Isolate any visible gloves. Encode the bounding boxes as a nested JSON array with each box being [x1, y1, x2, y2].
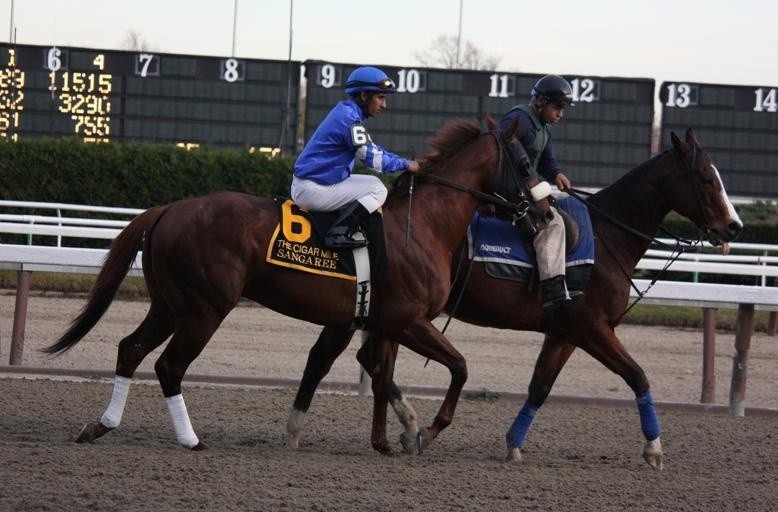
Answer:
[[518, 157, 530, 177]]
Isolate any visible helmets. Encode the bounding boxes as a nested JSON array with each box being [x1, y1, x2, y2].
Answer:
[[343, 66, 397, 94], [531, 76, 573, 102]]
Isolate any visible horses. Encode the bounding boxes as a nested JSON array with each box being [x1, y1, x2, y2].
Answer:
[[37, 114, 554, 452], [285, 125, 745, 474]]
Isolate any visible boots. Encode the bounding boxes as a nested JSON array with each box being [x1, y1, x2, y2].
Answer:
[[541, 277, 584, 312], [324, 200, 370, 252]]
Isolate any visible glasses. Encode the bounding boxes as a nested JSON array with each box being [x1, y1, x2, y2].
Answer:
[[378, 79, 397, 90]]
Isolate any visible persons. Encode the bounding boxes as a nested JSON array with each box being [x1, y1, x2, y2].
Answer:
[[482, 76, 587, 317], [290, 66, 422, 254]]
